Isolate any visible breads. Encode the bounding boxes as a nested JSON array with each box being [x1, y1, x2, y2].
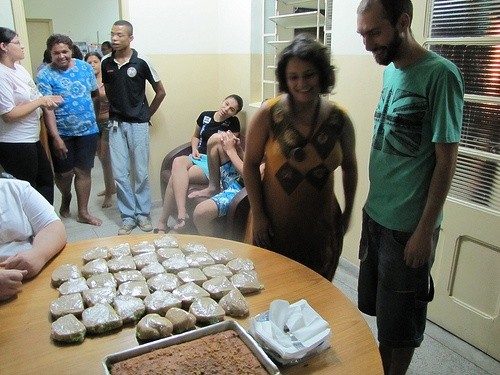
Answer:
[[112, 329, 271, 375], [49, 237, 263, 343]]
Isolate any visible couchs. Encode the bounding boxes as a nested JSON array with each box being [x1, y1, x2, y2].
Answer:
[[160, 141, 250, 242]]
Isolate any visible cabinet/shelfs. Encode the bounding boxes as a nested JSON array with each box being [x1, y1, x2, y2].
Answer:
[[246, 0, 333, 144]]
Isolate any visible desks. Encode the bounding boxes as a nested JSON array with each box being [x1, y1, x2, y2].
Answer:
[[0, 234, 385, 375]]
